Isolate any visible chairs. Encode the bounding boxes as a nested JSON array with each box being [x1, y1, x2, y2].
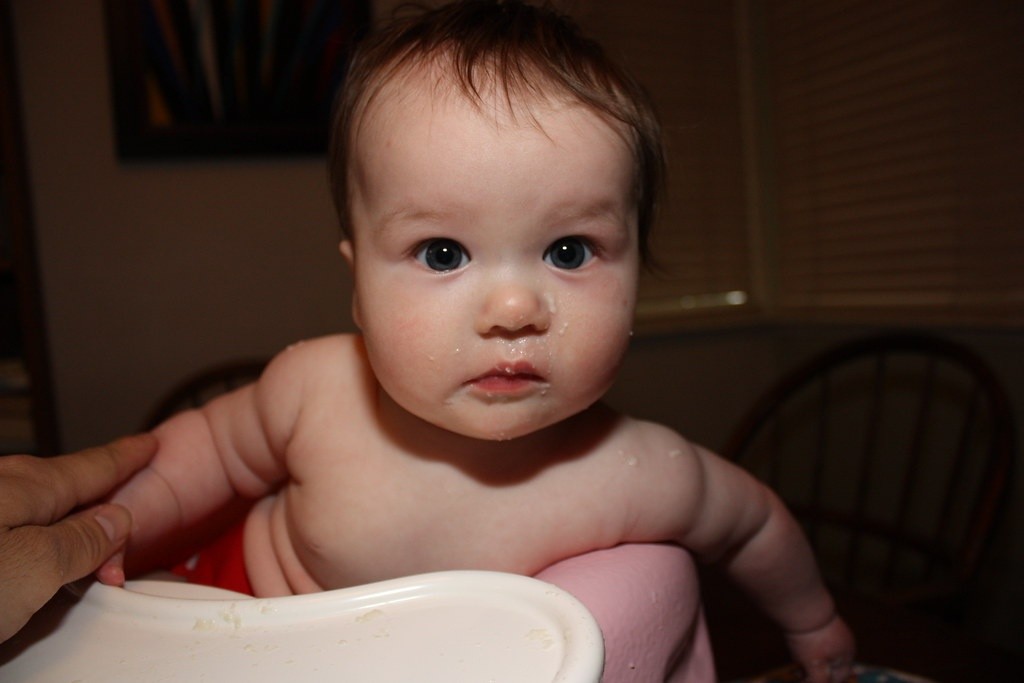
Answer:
[[718, 327, 1017, 616]]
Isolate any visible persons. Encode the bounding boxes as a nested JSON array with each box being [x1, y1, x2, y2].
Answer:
[[0, 433, 702, 683], [94, 0, 856, 683]]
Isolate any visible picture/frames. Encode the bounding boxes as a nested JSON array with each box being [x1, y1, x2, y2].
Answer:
[[103, 0, 369, 159]]
